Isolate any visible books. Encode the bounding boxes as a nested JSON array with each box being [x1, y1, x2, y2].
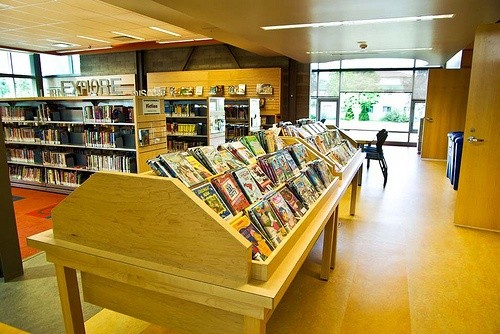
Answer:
[[224, 100, 250, 142], [162, 99, 209, 153], [0, 98, 137, 188], [146, 118, 358, 261], [158, 84, 273, 97]]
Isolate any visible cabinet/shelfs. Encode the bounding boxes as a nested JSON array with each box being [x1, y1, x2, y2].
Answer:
[[26, 135, 341, 333], [0, 93, 167, 195], [0, 116, 24, 283], [265, 124, 368, 271], [162, 96, 226, 153], [260, 112, 282, 131], [222, 97, 262, 143]]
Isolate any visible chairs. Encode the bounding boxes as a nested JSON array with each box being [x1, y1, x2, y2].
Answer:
[[354, 128, 389, 188]]
